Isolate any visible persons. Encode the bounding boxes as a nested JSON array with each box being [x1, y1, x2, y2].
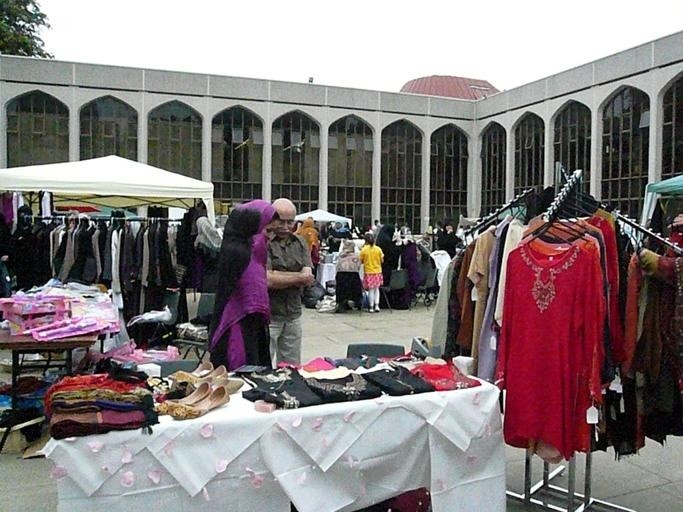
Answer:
[[208, 199, 316, 372], [360, 234, 384, 313], [266, 197, 315, 367], [298, 218, 320, 279], [194, 216, 223, 292], [437, 223, 459, 258]]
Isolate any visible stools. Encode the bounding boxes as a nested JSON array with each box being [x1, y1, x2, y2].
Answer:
[[172, 339, 206, 366]]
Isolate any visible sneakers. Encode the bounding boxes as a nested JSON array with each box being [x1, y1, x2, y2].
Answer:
[[369, 307, 380, 312]]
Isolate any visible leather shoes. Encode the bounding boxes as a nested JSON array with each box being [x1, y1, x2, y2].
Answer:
[[158, 361, 244, 420]]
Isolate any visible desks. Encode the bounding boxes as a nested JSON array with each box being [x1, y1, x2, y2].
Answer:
[[314, 263, 365, 289], [51, 358, 506, 512], [0, 332, 98, 411]]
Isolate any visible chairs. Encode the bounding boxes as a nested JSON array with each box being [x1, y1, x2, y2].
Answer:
[[414, 269, 438, 308], [133, 291, 180, 348], [379, 270, 412, 313], [348, 344, 405, 359]]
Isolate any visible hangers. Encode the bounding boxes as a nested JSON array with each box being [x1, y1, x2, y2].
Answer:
[[527, 173, 598, 247], [19, 213, 180, 233], [619, 215, 671, 258], [461, 193, 527, 251]]
